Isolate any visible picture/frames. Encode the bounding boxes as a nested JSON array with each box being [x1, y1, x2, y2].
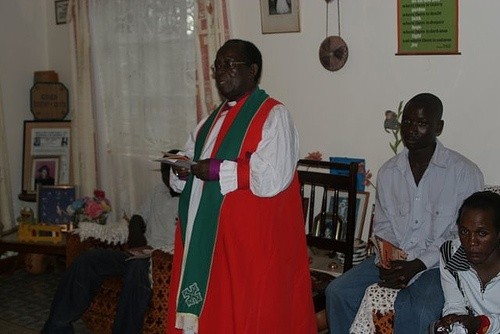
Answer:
[[22, 120, 72, 199], [318, 190, 371, 246], [29, 83, 70, 121], [36, 184, 75, 233], [261, 0, 301, 36], [28, 154, 61, 194], [54, 0, 70, 26]]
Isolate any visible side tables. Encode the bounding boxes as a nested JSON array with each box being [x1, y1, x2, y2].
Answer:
[[0, 225, 73, 281]]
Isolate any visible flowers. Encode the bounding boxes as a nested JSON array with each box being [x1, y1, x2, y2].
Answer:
[[66, 190, 112, 224]]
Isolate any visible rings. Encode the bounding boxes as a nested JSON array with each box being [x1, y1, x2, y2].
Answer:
[[176, 172, 178, 176]]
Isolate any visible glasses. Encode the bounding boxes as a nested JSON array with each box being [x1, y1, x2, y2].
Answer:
[[210, 61, 246, 75]]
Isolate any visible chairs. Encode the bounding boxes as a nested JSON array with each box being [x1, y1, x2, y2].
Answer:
[[349, 184, 500, 334], [294, 157, 358, 330]]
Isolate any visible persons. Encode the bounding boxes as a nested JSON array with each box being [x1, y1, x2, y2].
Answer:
[[430, 190, 500, 334], [325, 93, 484, 334], [34, 165, 54, 192], [41, 149, 182, 334], [165, 38, 317, 334]]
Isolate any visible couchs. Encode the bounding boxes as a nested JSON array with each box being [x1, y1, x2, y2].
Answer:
[[64, 186, 178, 334]]
